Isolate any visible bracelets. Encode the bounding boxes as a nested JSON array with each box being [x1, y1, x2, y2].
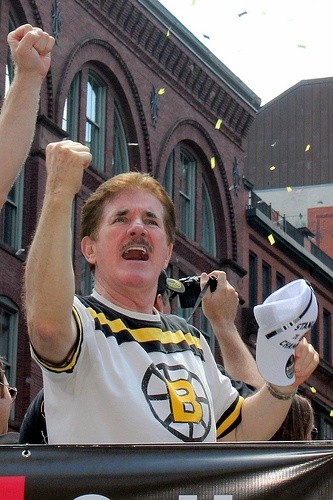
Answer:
[[268, 382, 299, 400]]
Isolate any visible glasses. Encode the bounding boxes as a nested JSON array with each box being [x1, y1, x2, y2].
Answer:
[[0, 382, 18, 402]]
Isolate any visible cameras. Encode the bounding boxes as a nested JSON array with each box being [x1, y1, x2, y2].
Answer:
[[178, 275, 217, 308]]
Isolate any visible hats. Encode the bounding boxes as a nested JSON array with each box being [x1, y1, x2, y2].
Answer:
[[253, 278, 318, 387], [157, 269, 185, 294]]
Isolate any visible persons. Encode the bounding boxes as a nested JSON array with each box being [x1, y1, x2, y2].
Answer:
[[153, 268, 266, 398], [19, 388, 48, 444], [0, 361, 16, 436], [0, 22, 55, 208], [22, 139, 320, 445], [270, 395, 315, 441]]
